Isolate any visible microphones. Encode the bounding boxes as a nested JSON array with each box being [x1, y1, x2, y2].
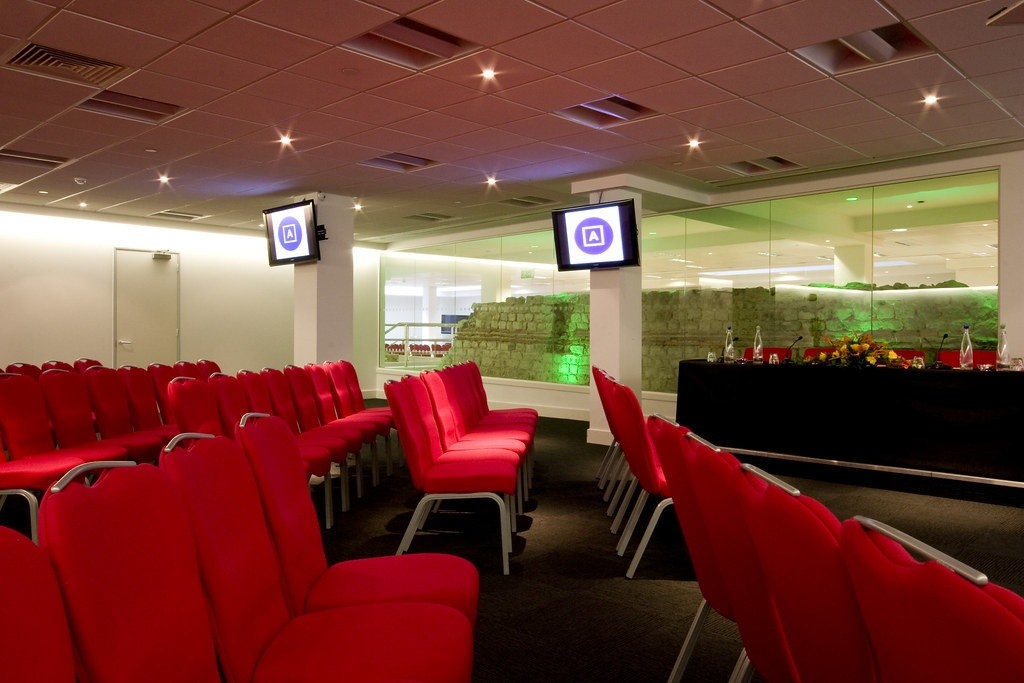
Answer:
[[719, 337, 739, 363], [932, 334, 952, 370], [782, 336, 803, 364]]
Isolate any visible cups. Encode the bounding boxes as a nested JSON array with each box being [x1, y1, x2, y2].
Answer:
[[707, 352, 717, 363], [1011, 358, 1023, 371], [769, 353, 779, 364]]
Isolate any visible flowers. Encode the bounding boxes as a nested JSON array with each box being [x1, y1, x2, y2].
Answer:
[[803, 331, 910, 370]]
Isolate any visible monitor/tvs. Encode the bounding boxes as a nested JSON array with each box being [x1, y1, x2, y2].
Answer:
[[551, 198, 642, 271], [263, 199, 328, 267]]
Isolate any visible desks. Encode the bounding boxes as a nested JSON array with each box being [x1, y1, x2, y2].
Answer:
[[677, 358, 1024, 510]]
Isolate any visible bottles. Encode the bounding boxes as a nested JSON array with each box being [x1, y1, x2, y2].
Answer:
[[996, 324, 1010, 371], [724, 326, 735, 364], [959, 324, 973, 370], [752, 326, 764, 364]]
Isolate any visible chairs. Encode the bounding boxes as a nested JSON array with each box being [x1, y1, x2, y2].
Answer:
[[1, 358, 1023, 681]]
